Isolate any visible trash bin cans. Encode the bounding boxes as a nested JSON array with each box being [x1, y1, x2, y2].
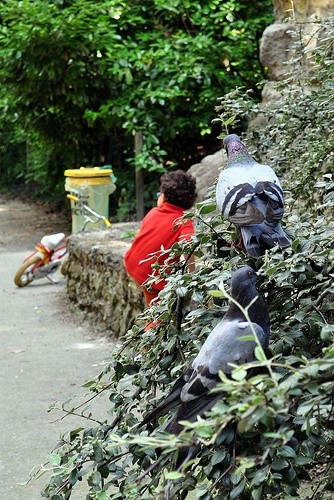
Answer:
[[64, 165, 117, 234]]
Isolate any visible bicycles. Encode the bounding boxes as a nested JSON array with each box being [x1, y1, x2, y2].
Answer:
[[13, 194, 111, 287]]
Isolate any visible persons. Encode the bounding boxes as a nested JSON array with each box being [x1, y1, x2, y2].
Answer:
[[122, 170, 198, 334]]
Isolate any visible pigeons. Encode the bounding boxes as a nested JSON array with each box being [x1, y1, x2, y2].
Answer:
[[136, 267, 275, 437], [215, 134, 291, 259]]
